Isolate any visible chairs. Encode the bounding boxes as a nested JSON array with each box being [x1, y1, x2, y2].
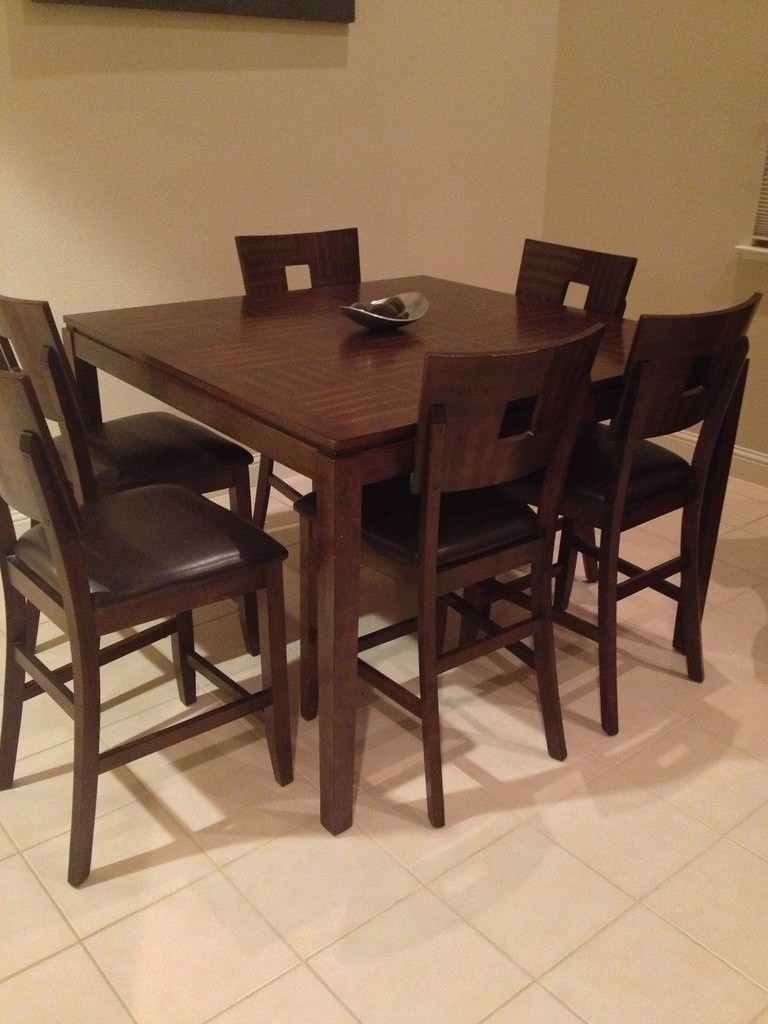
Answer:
[[0, 370, 291, 886], [292, 323, 605, 829], [0, 297, 261, 657], [231, 226, 362, 529], [506, 237, 636, 586], [478, 293, 762, 736]]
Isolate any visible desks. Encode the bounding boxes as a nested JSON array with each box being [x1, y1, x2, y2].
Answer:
[[61, 273, 640, 837]]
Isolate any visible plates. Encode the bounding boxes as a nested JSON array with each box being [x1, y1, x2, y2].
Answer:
[[339, 292, 429, 330]]
